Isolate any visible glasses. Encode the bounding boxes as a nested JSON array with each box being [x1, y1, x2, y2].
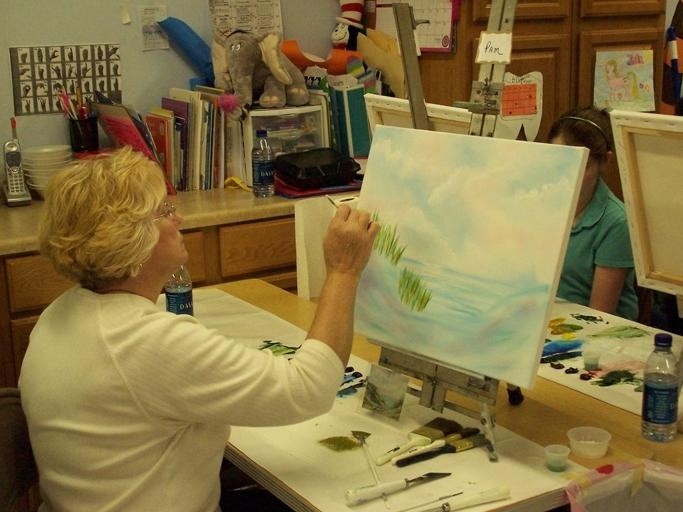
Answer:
[[150, 199, 176, 222]]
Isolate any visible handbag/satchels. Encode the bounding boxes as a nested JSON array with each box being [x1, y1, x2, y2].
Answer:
[[276, 147, 355, 188]]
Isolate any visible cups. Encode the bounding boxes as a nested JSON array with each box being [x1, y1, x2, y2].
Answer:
[[543, 443, 570, 472], [582, 352, 602, 370]]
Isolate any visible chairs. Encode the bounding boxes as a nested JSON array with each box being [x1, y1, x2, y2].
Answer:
[[0, 387, 41, 510]]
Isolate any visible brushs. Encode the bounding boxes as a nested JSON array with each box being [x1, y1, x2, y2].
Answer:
[[377, 417, 488, 467]]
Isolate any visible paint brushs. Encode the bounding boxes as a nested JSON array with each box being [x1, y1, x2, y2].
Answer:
[[351, 430, 388, 501], [325, 195, 339, 209]]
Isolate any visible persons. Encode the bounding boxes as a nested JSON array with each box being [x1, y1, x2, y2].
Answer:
[[539, 106, 644, 322], [15, 141, 382, 512]]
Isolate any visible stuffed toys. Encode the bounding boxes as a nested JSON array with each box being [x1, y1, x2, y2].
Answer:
[[324, 0, 367, 65], [210, 24, 311, 108]]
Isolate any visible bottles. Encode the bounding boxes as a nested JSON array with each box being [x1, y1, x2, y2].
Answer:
[[251, 130, 275, 197], [163, 265, 193, 319], [640, 332, 679, 443]]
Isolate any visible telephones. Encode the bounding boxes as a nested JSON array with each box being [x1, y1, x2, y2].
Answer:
[[0, 116, 32, 207]]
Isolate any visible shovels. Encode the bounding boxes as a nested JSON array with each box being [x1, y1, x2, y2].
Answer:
[[347, 471, 453, 505]]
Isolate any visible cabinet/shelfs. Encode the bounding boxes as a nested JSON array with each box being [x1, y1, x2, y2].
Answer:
[[379, 1, 667, 201], [0, 186, 301, 392]]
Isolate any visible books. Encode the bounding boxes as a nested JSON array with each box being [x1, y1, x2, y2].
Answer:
[[97, 78, 385, 199]]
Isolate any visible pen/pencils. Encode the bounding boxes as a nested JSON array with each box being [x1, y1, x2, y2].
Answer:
[[58, 82, 91, 152]]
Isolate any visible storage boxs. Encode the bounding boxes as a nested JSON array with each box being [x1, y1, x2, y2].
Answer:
[[228, 94, 331, 194]]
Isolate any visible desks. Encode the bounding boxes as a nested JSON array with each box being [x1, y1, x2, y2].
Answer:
[[310, 290, 681, 477], [148, 276, 657, 512]]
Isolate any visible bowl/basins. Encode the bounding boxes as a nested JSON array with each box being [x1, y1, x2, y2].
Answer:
[[20, 143, 74, 200], [566, 425, 611, 461]]
